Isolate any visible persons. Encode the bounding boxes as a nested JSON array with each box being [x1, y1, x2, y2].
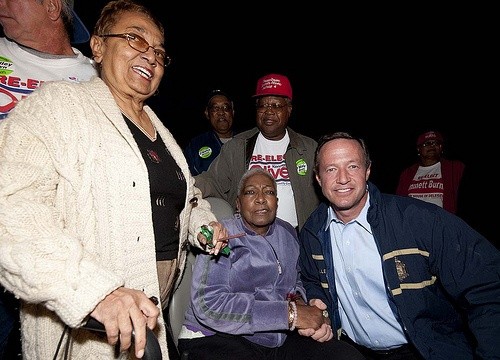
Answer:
[[0, 0, 102, 123], [300, 131, 500, 360], [0, 0, 230, 360], [194, 74, 319, 239], [396, 131, 475, 217], [182, 89, 235, 176], [177, 169, 365, 360]]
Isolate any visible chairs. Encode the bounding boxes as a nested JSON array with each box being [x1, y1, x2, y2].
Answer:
[[169, 196, 233, 345]]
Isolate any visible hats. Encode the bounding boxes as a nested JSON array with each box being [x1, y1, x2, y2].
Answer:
[[416, 132, 443, 146], [253, 74, 293, 100], [63, 0, 91, 44]]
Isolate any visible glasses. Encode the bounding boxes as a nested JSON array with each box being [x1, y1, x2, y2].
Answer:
[[255, 103, 289, 113], [99, 32, 172, 68], [421, 141, 441, 147], [208, 105, 234, 113]]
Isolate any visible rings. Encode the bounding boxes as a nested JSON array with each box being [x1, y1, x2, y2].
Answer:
[[323, 310, 328, 317]]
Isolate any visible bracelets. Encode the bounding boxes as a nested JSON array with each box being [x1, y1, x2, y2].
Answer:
[[288, 302, 297, 331]]
